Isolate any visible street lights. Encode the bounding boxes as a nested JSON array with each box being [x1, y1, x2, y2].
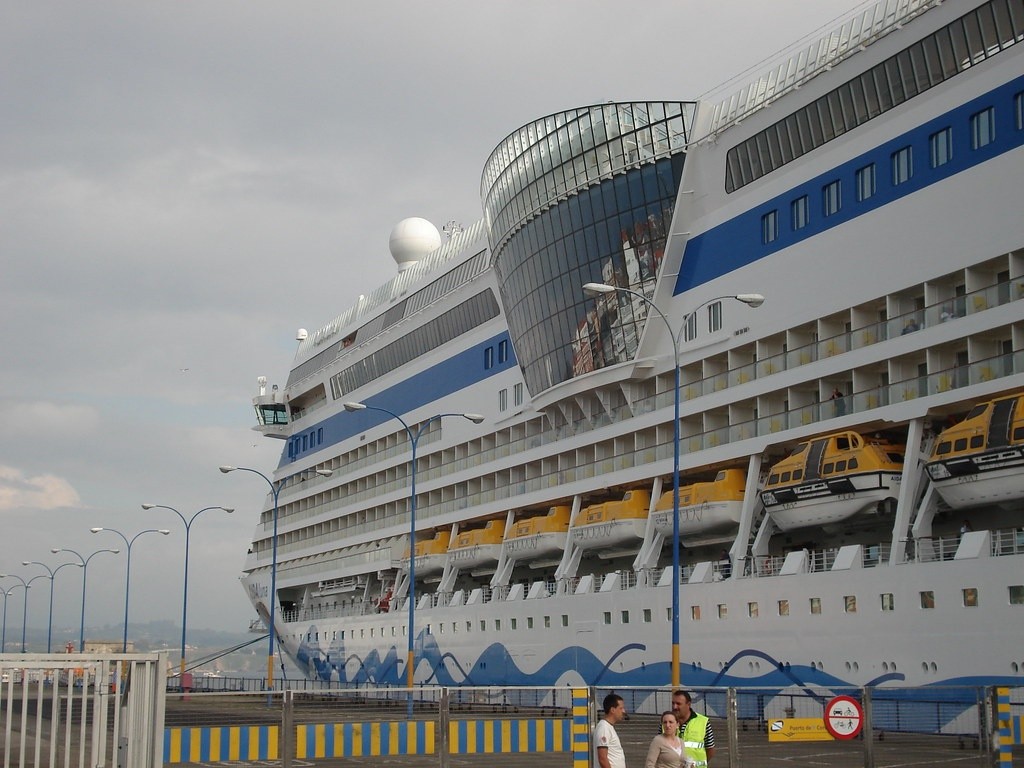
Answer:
[[343, 400, 486, 719], [0, 584, 32, 653], [90, 527, 170, 654], [22, 560, 84, 653], [52, 548, 120, 655], [1, 573, 54, 653], [218, 465, 333, 703], [141, 501, 235, 703], [581, 281, 764, 722]]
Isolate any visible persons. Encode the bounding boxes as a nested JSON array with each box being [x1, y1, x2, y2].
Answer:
[[645, 711, 694, 768], [902, 319, 919, 335], [941, 307, 959, 321], [593, 694, 626, 768], [830, 387, 846, 417], [658, 690, 716, 768]]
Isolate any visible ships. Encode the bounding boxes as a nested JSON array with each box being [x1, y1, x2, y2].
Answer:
[[236, 3, 1024, 749]]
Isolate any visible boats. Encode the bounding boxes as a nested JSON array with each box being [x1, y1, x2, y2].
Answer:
[[501, 506, 580, 572], [763, 432, 907, 536], [651, 466, 748, 548], [568, 488, 653, 559], [401, 530, 451, 585], [921, 392, 1024, 513], [445, 516, 507, 579]]
